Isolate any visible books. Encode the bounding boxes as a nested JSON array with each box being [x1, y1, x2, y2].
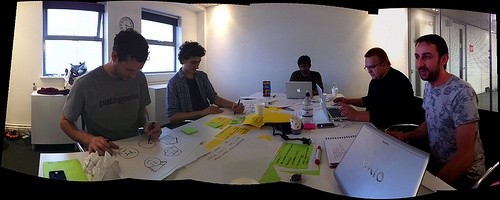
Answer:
[[325, 135, 357, 168]]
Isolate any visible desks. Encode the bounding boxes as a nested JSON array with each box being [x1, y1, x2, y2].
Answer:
[[144, 83, 168, 123], [38, 92, 457, 199], [30, 88, 83, 151]]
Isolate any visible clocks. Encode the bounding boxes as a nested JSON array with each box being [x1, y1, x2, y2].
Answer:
[[119, 17, 134, 32]]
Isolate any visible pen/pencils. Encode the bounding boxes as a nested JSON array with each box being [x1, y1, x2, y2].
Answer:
[[148, 122, 155, 142], [99, 136, 112, 156], [234, 99, 240, 114]]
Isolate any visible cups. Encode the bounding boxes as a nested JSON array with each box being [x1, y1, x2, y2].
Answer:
[[253, 103, 265, 116], [289, 112, 301, 135]]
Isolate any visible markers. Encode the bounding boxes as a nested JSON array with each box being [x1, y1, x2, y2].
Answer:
[[315, 146, 321, 164]]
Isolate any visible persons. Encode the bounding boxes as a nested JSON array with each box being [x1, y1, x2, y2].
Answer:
[[166, 40, 245, 128], [64, 68, 70, 89], [386, 34, 485, 189], [59, 28, 162, 157], [333, 48, 416, 132], [289, 55, 324, 96]]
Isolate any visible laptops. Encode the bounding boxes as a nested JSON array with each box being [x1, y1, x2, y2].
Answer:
[[334, 123, 456, 200], [285, 82, 312, 99], [316, 84, 352, 121]]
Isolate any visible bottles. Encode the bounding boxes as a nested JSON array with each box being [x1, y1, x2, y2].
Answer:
[[302, 92, 313, 124], [331, 81, 339, 96]]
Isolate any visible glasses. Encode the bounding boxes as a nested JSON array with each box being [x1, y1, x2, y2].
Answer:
[[364, 60, 385, 72]]
